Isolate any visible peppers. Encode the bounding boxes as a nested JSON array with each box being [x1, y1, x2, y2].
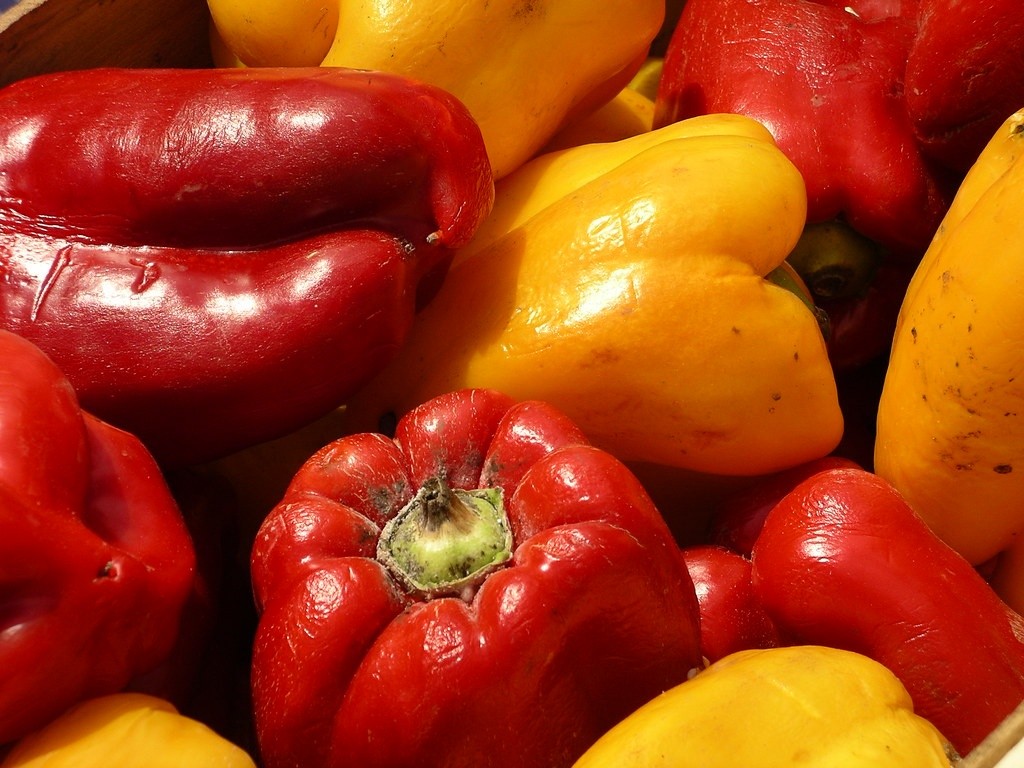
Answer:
[[0, 0, 1024, 768]]
